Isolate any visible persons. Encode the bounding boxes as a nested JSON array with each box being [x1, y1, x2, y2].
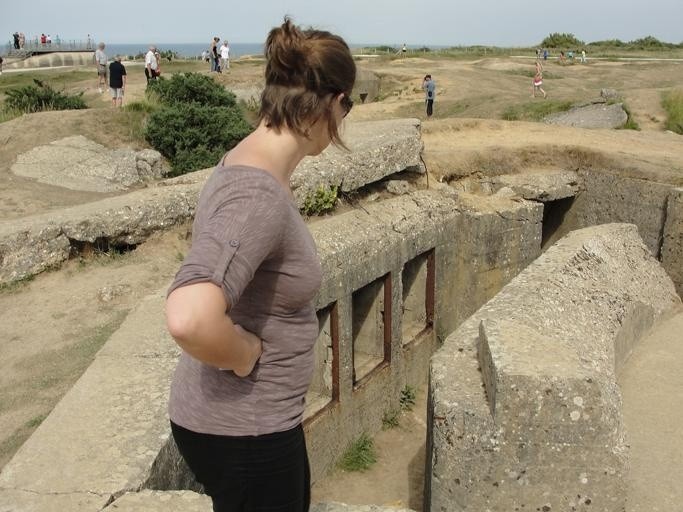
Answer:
[[144, 46, 157, 83], [95, 43, 109, 93], [529, 60, 547, 98], [536, 45, 587, 65], [109, 55, 127, 106], [200, 35, 230, 72], [155, 51, 161, 79], [163, 14, 358, 512], [402, 43, 407, 58], [0, 31, 94, 74], [422, 74, 436, 120]]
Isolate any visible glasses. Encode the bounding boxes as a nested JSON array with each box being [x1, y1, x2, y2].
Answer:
[[322, 84, 353, 118]]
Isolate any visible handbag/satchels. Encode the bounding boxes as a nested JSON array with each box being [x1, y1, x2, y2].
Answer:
[[534, 78, 543, 86], [429, 92, 432, 97]]
